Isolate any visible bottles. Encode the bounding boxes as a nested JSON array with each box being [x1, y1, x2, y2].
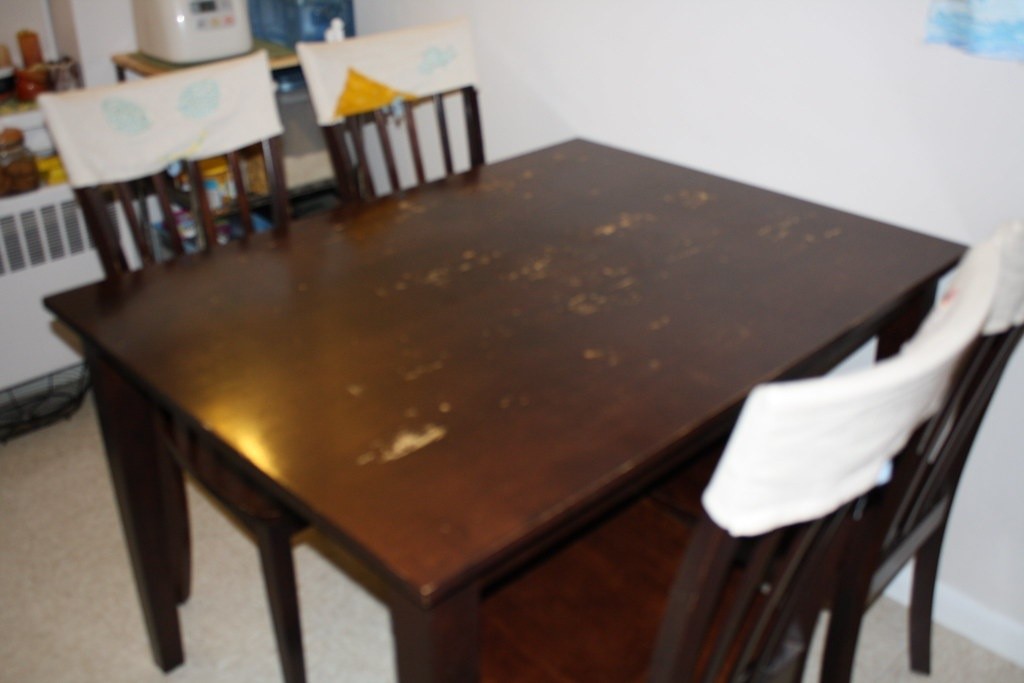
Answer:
[[0, 132, 38, 197]]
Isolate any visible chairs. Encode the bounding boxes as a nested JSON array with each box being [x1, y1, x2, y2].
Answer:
[[297, 17, 484, 202], [817, 224, 1024, 683], [36, 49, 360, 683], [481, 333, 880, 682]]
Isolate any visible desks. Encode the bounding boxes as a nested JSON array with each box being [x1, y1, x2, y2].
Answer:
[[111, 40, 300, 82], [44, 139, 968, 682]]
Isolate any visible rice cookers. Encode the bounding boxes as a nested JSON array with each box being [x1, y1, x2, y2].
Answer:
[[130, 0, 252, 64]]
